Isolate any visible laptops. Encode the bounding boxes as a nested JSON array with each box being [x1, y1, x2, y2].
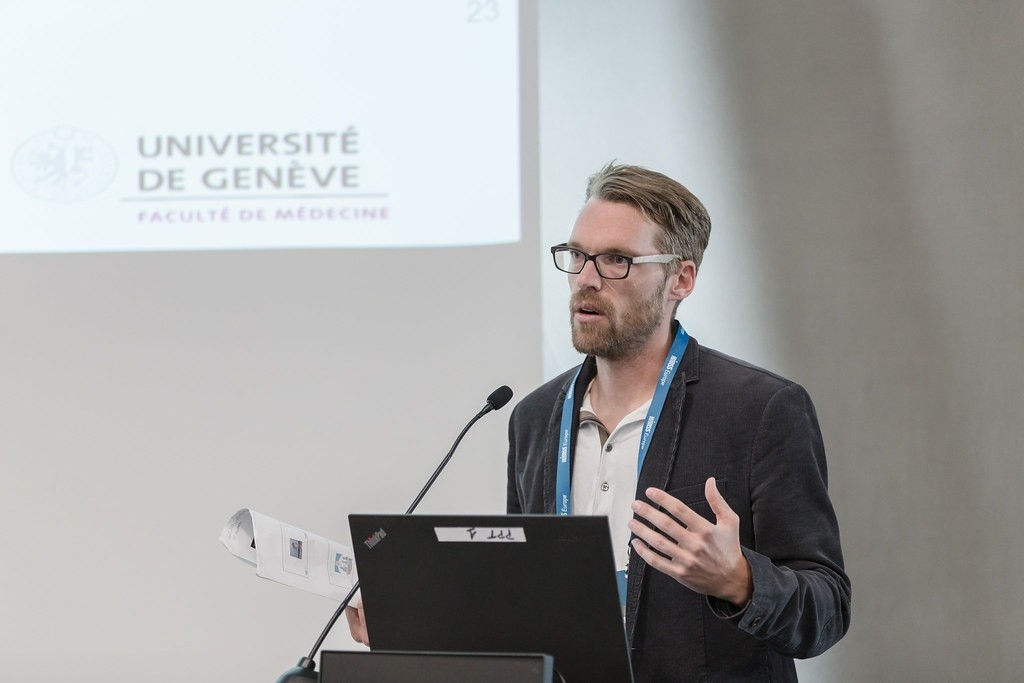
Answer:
[[347, 514, 634, 683]]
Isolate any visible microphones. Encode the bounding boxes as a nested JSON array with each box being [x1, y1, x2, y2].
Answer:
[[278, 385, 514, 683]]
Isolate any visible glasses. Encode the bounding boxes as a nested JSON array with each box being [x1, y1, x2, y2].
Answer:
[[551, 243, 681, 280]]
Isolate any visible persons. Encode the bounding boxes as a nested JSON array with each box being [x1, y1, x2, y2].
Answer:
[[346, 163, 853, 682]]
